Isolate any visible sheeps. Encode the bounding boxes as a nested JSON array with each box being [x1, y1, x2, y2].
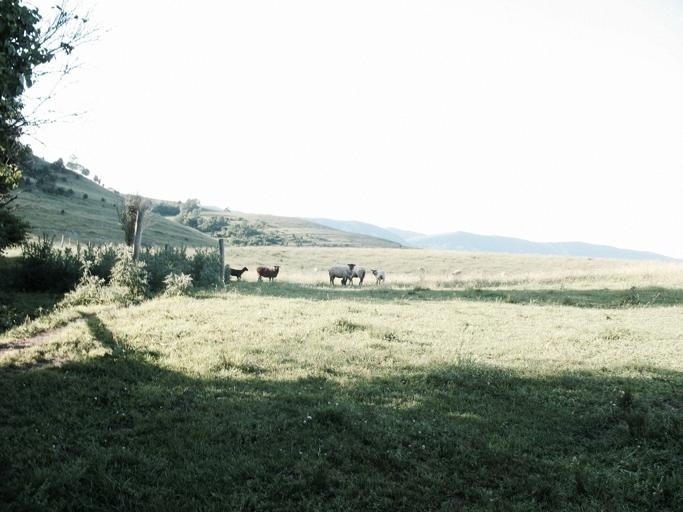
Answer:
[[256, 264, 280, 282], [328, 263, 386, 289], [229, 267, 248, 281]]
